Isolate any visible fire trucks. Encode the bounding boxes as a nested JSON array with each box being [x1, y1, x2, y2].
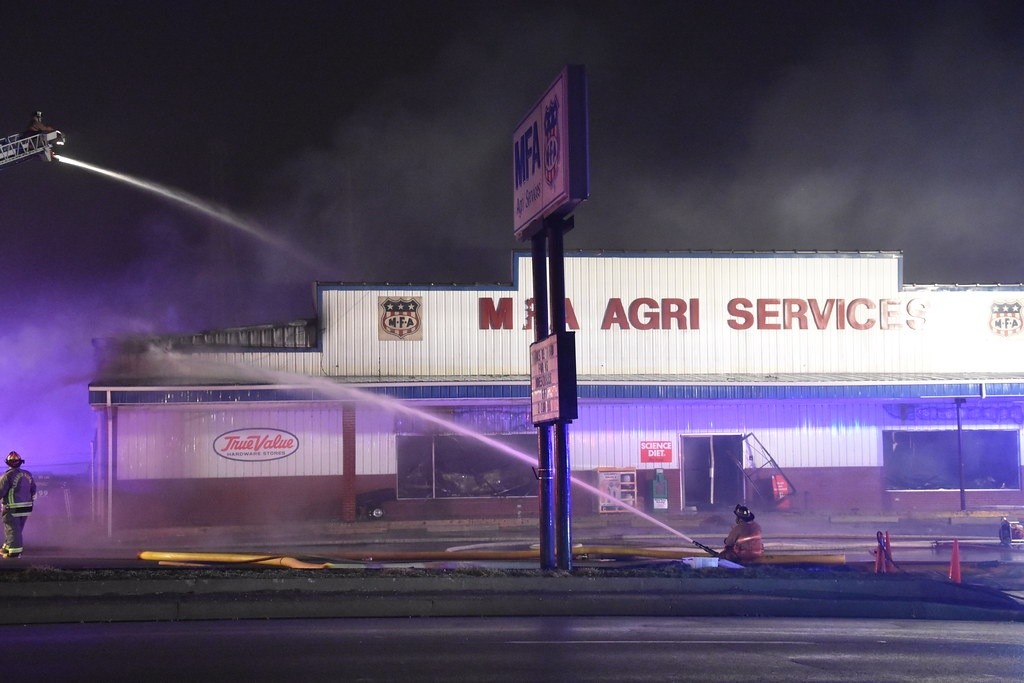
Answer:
[[0, 130, 69, 171]]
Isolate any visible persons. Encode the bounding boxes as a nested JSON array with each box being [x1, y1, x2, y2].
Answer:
[[0, 451, 36, 557], [718, 505, 764, 564]]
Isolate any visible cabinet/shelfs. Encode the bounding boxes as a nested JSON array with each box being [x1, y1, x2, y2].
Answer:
[[592, 467, 638, 514]]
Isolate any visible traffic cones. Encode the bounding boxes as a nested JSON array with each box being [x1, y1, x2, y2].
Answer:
[[947, 536, 961, 584], [875, 532, 885, 576], [883, 530, 900, 575]]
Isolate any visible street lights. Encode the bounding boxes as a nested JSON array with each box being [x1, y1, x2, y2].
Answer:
[[952, 396, 967, 513]]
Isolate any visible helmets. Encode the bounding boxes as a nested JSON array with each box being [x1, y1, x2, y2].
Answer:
[[734, 506, 755, 522], [4, 450, 22, 468]]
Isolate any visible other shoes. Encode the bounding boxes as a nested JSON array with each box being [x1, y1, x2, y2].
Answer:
[[0, 548, 9, 558]]
